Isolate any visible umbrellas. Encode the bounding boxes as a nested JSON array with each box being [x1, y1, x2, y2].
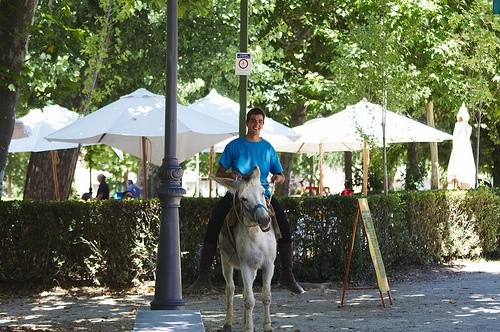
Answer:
[[8, 104, 102, 200], [297, 98, 453, 197], [44, 87, 237, 201], [263, 112, 389, 196], [445, 102, 479, 189], [185, 90, 307, 200]]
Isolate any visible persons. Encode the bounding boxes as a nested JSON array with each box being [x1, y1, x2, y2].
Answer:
[[341, 182, 353, 197], [96, 174, 110, 201], [322, 187, 331, 197], [195, 107, 305, 294], [128, 180, 141, 198], [123, 191, 133, 199]]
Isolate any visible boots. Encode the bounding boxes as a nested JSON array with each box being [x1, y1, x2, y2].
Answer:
[[188, 241, 217, 290], [278, 243, 305, 295]]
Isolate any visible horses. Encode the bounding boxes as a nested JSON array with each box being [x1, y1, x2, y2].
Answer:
[[211, 165, 278, 332]]
